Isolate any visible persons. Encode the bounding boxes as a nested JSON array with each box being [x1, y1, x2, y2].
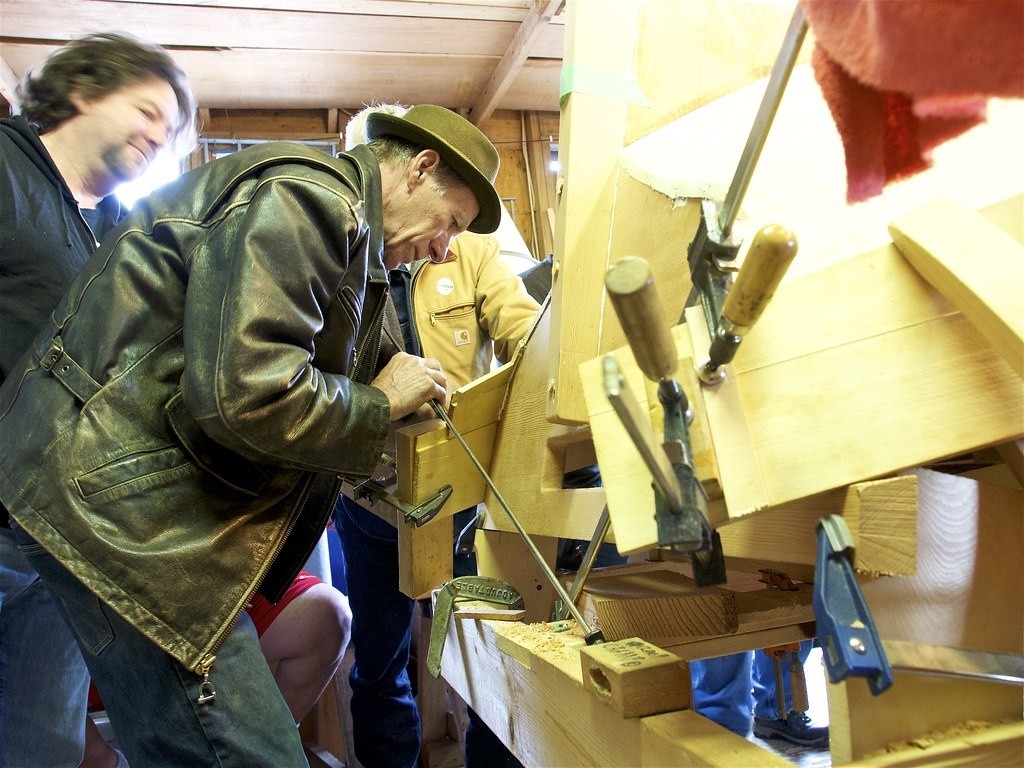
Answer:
[[0, 104, 503, 768], [0, 32, 554, 768]]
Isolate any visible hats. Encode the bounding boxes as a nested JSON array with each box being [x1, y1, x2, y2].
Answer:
[[367, 103, 500, 233]]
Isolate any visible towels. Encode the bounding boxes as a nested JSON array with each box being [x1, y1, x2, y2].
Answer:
[[794, 1, 1024, 205]]
